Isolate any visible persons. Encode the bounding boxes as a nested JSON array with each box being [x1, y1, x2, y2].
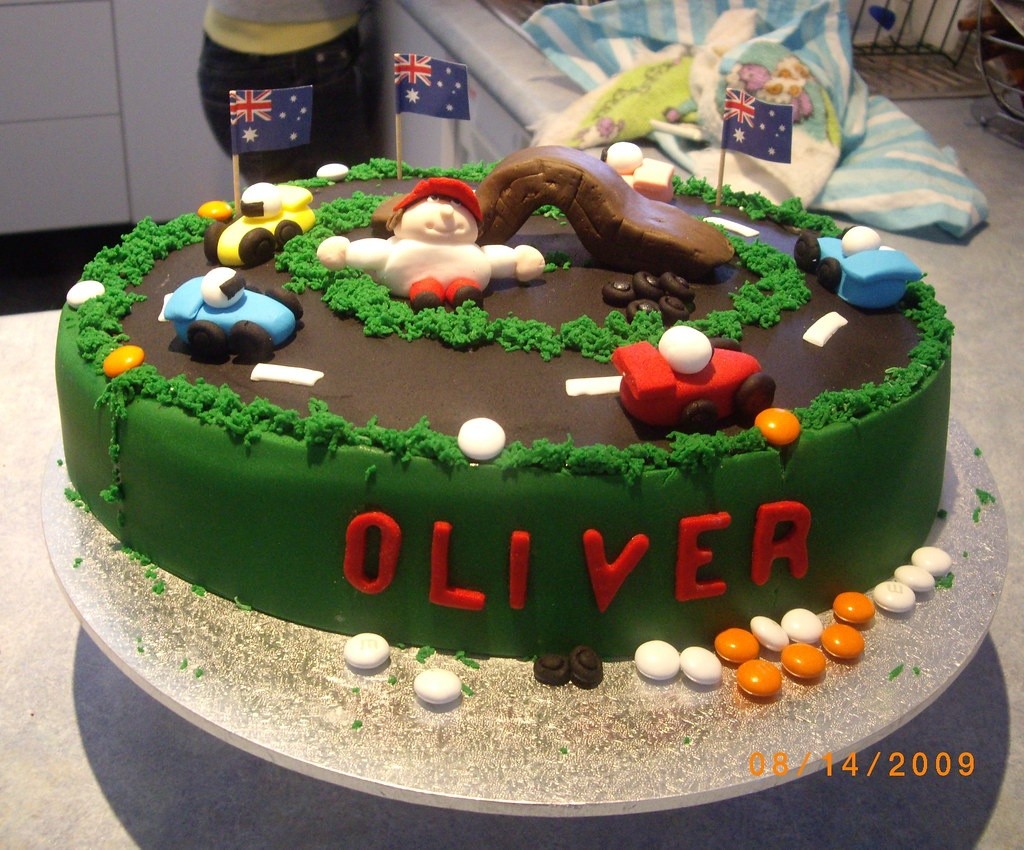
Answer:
[[197, 0, 382, 188], [317, 179, 545, 312]]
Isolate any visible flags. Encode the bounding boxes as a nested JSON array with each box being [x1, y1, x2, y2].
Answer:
[[722, 88, 793, 164], [229, 86, 311, 152], [394, 53, 470, 120]]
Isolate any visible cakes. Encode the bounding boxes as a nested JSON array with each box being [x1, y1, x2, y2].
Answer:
[[52, 50, 955, 702]]
